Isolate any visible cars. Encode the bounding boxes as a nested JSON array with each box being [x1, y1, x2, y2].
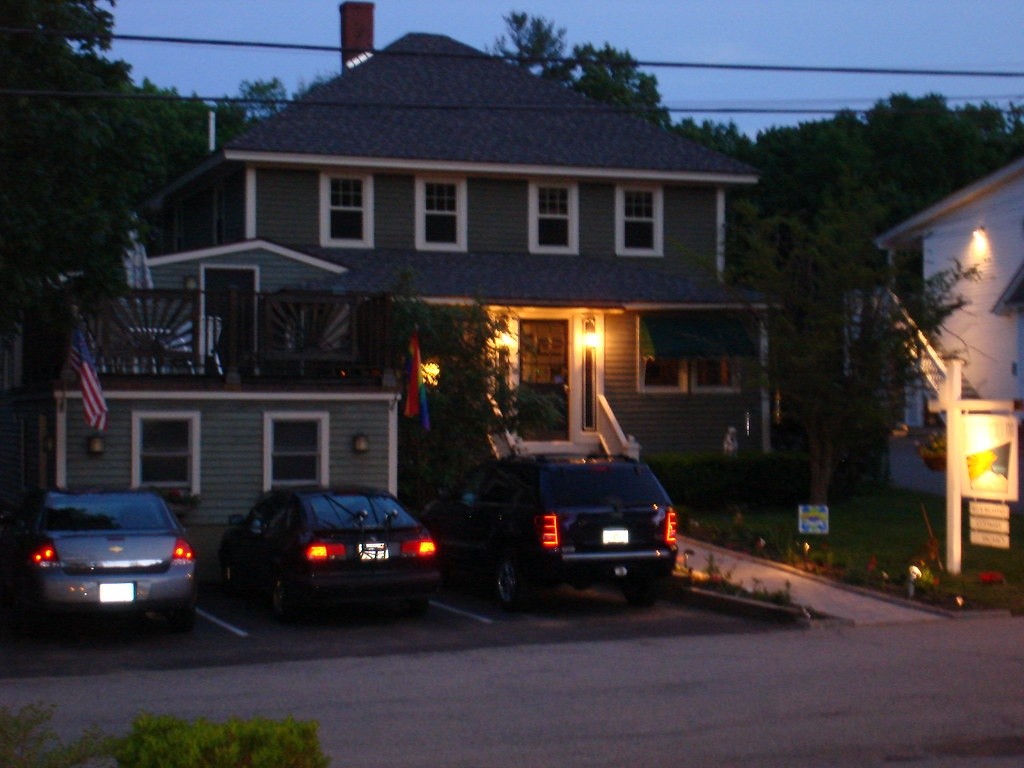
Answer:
[[214, 484, 439, 622], [5, 489, 199, 636]]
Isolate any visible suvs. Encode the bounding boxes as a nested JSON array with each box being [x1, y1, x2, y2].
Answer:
[[417, 454, 676, 616]]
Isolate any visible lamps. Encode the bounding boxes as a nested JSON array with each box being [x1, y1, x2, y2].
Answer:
[[87, 430, 106, 455], [352, 433, 371, 452], [579, 310, 599, 346]]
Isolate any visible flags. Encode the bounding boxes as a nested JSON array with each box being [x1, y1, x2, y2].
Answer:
[[399, 329, 433, 431], [66, 314, 110, 433]]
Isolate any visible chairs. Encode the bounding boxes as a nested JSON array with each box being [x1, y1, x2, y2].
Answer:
[[152, 315, 222, 375]]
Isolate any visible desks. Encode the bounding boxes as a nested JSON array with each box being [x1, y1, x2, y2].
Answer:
[[122, 325, 172, 373]]
